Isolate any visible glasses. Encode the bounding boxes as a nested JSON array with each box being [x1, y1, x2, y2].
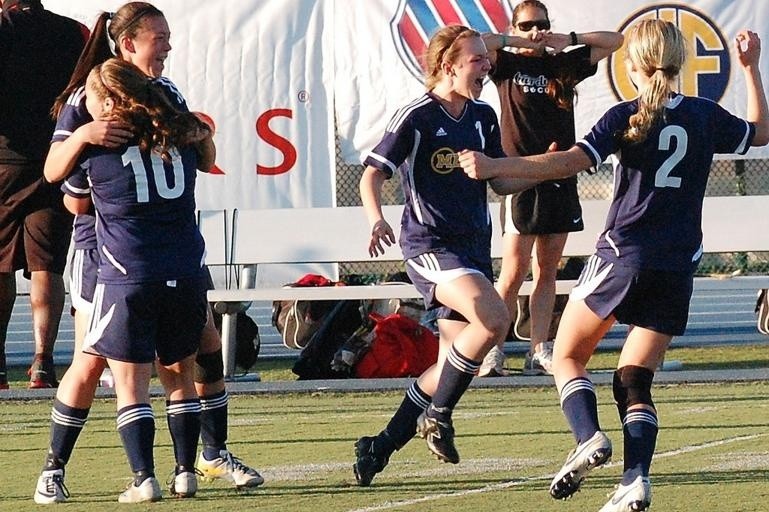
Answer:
[[515, 20, 551, 32]]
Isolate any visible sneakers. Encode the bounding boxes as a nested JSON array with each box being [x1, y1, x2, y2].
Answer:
[[118, 474, 162, 503], [417, 405, 461, 464], [547, 432, 611, 500], [599, 475, 651, 512], [522, 342, 554, 376], [352, 434, 391, 486], [197, 450, 264, 487], [479, 344, 505, 376], [166, 466, 205, 497], [28, 353, 57, 387], [33, 469, 70, 504]]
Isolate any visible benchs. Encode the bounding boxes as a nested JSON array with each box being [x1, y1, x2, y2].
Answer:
[[1, 194, 766, 390]]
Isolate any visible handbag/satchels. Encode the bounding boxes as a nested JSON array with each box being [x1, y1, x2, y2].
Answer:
[[273, 275, 440, 379], [510, 258, 584, 340], [234, 312, 261, 370]]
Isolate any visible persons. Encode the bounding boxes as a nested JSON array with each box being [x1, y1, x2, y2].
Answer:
[[458, 17, 768, 512], [62, 57, 215, 505], [478, 0, 624, 377], [0, 0, 91, 389], [354, 24, 564, 486], [32, 1, 266, 507]]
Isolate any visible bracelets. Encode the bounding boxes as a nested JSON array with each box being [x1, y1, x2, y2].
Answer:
[[500, 34, 507, 49], [569, 31, 579, 46]]
